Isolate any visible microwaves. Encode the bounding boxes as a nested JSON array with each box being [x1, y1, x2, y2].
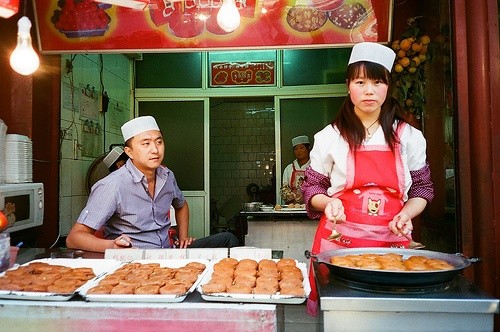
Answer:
[[1, 182, 44, 232]]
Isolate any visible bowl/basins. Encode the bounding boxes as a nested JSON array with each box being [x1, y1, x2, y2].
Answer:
[[242, 201, 274, 212]]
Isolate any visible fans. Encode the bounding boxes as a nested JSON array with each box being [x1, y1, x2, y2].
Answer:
[[246, 183, 259, 202]]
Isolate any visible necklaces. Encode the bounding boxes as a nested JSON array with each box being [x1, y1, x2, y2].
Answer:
[[366, 118, 379, 135]]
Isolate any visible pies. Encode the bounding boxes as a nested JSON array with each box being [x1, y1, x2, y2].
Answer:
[[202, 258, 306, 295], [331, 254, 455, 270], [0, 261, 95, 293], [87, 261, 205, 296]]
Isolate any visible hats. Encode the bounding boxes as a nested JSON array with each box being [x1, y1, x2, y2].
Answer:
[[121, 116, 161, 142], [292, 136, 311, 147], [348, 42, 397, 73], [103, 146, 125, 168]]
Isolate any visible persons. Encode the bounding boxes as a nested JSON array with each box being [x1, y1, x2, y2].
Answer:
[[301, 42, 434, 317], [66, 116, 238, 253], [283, 135, 312, 207]]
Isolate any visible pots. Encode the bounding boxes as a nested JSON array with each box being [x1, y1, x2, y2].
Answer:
[[303, 244, 482, 290]]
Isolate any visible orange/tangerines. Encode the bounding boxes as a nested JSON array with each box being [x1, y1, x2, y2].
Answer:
[[392, 33, 430, 106]]
